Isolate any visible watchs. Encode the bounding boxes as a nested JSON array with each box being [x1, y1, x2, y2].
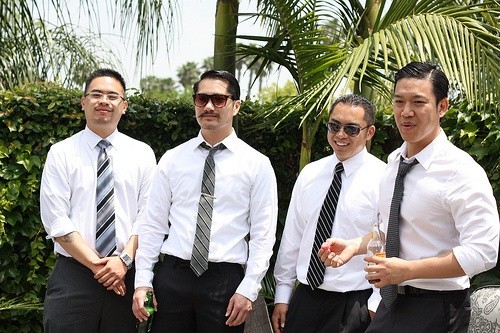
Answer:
[[118, 251, 133, 270]]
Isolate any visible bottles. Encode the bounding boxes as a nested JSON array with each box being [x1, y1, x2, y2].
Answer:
[[366, 223, 386, 284], [136, 292, 155, 333]]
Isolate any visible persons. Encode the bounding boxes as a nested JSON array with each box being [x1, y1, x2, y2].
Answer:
[[273, 93, 388, 333], [133, 68, 278, 333], [40, 68, 157, 333], [318, 61, 500, 333]]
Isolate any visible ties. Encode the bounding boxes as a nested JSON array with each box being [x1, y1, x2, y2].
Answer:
[[305, 163, 345, 291], [95, 139, 118, 260], [189, 143, 228, 279], [379, 153, 418, 309]]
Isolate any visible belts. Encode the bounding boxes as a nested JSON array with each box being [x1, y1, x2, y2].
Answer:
[[398, 285, 469, 297]]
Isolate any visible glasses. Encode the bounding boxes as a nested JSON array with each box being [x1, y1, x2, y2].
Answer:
[[84, 91, 126, 101], [191, 92, 233, 107], [326, 120, 372, 136]]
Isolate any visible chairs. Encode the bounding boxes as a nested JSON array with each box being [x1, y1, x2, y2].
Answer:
[[468, 285, 500, 333]]
